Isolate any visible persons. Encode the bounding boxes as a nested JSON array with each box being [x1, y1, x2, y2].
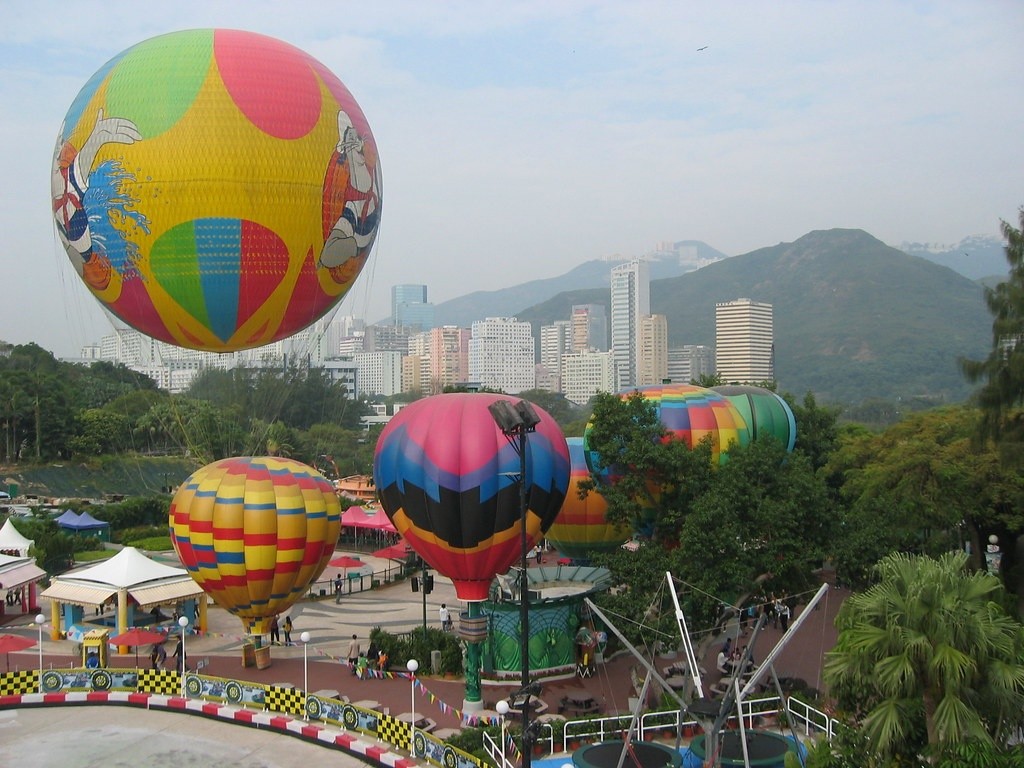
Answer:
[[347, 634, 387, 681], [5, 586, 22, 607], [85, 651, 99, 669], [715, 590, 791, 679], [172, 634, 187, 672], [334, 574, 343, 604], [194, 603, 201, 629], [439, 604, 450, 633], [270, 613, 281, 646], [149, 605, 166, 626], [148, 642, 166, 670], [282, 616, 294, 647]]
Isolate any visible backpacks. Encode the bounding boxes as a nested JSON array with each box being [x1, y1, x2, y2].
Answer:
[[335, 582, 341, 592]]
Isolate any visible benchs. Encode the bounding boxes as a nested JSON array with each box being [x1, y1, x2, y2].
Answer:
[[567, 706, 600, 717], [717, 664, 759, 677], [663, 660, 707, 679], [342, 695, 350, 704], [383, 707, 390, 715], [423, 717, 437, 732], [396, 713, 425, 726], [371, 704, 382, 710]]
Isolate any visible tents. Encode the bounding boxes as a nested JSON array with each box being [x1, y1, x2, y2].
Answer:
[[53, 509, 110, 547], [341, 505, 400, 552]]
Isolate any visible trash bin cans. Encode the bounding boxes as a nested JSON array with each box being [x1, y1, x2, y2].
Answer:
[[431, 650, 442, 676]]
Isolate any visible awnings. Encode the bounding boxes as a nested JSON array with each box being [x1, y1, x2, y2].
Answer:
[[38, 580, 117, 610], [0, 560, 47, 592], [126, 578, 207, 609]]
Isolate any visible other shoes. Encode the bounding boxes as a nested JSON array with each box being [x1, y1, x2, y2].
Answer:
[[336, 602, 341, 605], [352, 671, 355, 676], [750, 626, 755, 630], [761, 627, 765, 631]]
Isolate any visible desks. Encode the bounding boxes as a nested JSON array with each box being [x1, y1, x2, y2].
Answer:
[[667, 678, 685, 689], [728, 660, 752, 673], [270, 682, 294, 689], [567, 690, 594, 716], [314, 689, 341, 700], [673, 661, 697, 675], [432, 728, 463, 740], [352, 700, 379, 710]]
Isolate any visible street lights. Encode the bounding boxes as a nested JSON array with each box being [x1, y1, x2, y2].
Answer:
[[496, 701, 511, 768], [404, 659, 421, 761], [34, 614, 45, 693], [300, 631, 311, 720], [177, 615, 189, 699]]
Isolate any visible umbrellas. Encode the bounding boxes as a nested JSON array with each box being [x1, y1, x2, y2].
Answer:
[[106, 628, 166, 669], [0, 633, 37, 672], [330, 556, 366, 595], [371, 546, 410, 582]]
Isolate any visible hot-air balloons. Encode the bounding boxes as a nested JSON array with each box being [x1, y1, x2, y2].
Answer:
[[541, 433, 637, 565], [583, 378, 753, 556], [709, 384, 798, 490], [370, 391, 572, 721], [165, 453, 343, 670], [45, 25, 386, 474]]
